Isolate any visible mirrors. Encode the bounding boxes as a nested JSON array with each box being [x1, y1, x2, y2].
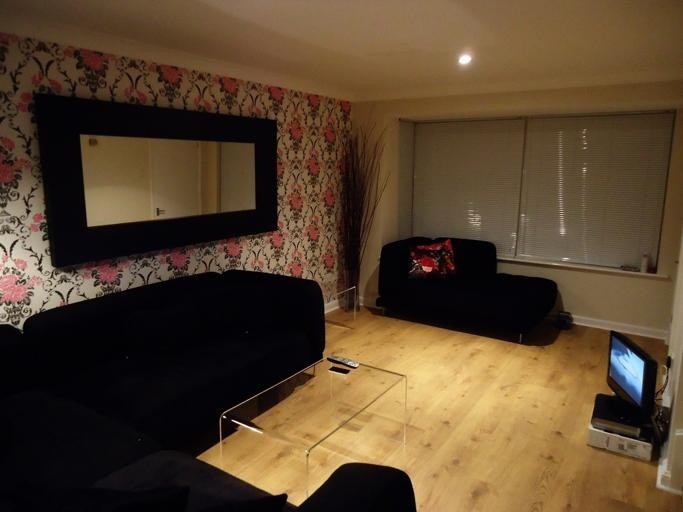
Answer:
[[34, 92, 278, 266]]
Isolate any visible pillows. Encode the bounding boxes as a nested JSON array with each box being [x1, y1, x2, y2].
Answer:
[[408, 240, 458, 282]]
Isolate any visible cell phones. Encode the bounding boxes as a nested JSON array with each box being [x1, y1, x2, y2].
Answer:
[[328, 366, 350, 376]]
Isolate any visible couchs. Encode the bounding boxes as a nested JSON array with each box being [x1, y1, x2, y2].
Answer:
[[374, 238, 575, 345], [0, 269, 418, 510]]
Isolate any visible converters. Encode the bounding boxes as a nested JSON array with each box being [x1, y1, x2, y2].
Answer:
[[666, 355, 672, 369]]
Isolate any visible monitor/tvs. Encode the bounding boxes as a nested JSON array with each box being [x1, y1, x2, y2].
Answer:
[[606, 329, 658, 419]]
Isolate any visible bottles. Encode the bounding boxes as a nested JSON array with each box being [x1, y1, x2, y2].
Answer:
[[640, 255, 648, 273]]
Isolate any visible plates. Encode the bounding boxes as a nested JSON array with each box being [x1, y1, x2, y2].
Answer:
[[623, 267, 639, 272]]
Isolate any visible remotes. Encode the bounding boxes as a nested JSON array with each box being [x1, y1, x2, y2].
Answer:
[[328, 354, 359, 368]]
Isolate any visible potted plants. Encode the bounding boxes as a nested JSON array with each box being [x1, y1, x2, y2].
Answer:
[[337, 124, 391, 313]]
